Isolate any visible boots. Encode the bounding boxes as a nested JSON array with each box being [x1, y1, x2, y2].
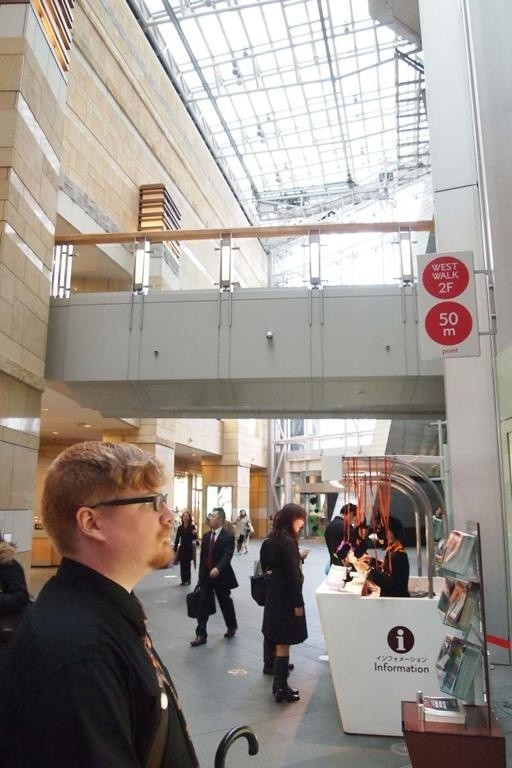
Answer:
[[271, 653, 300, 704]]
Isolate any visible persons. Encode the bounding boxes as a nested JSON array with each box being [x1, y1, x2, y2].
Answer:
[[0, 537, 33, 640], [345, 515, 412, 596], [172, 510, 198, 585], [232, 509, 253, 556], [261, 501, 309, 702], [1, 436, 202, 767], [323, 501, 361, 566], [250, 510, 312, 676], [188, 505, 239, 646]]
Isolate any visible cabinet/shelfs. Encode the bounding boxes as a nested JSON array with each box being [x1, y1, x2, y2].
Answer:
[[400, 519, 508, 768]]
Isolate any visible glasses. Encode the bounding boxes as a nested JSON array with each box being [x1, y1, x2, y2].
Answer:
[[85, 489, 170, 511]]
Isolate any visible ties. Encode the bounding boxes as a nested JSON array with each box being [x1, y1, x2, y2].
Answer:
[[206, 532, 216, 570]]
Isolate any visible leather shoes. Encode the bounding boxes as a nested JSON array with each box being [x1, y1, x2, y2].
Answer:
[[223, 624, 239, 638], [188, 635, 207, 646]]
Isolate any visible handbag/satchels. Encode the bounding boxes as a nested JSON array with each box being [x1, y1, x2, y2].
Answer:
[[249, 575, 268, 606], [184, 583, 217, 618]]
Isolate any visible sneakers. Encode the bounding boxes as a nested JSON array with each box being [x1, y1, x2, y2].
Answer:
[[181, 581, 191, 586], [260, 659, 295, 676]]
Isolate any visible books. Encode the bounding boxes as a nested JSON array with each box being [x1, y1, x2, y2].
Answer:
[[432, 527, 490, 708], [423, 694, 467, 726]]
